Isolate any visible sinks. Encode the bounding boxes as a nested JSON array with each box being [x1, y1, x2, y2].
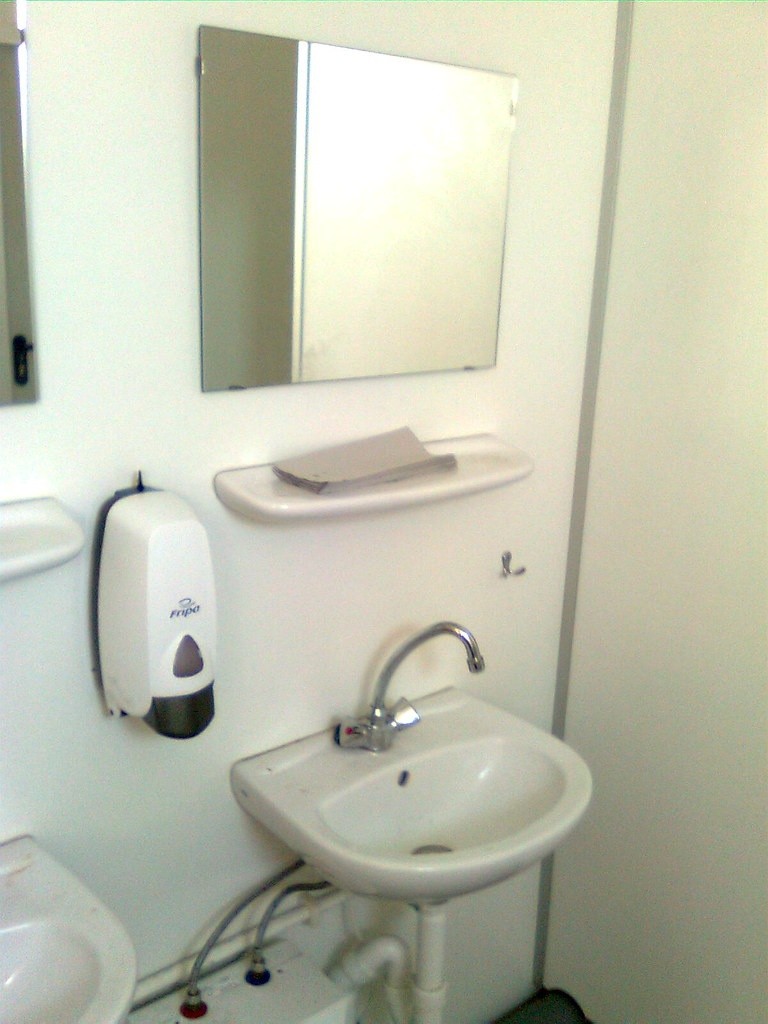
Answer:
[[231, 685, 593, 898], [0, 831, 138, 1022]]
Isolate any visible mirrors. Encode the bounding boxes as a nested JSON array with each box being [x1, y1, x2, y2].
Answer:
[[0, 0, 40, 409], [194, 23, 519, 391]]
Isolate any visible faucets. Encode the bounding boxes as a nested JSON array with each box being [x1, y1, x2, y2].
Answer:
[[336, 620, 486, 752]]
